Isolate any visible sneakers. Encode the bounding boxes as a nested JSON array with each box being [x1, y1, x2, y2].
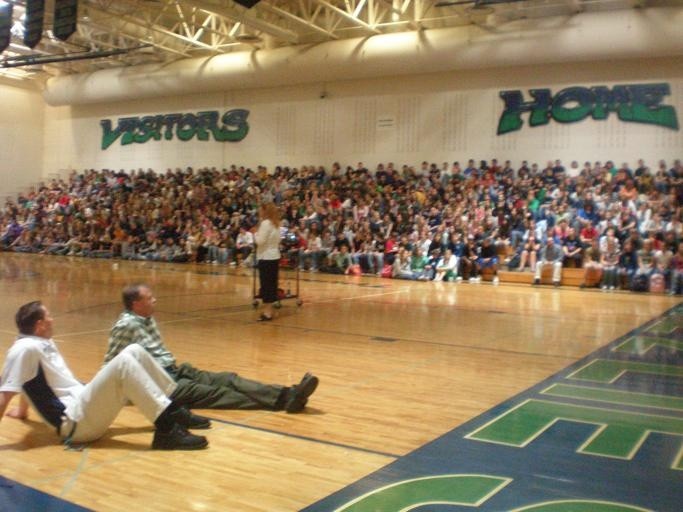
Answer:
[[170, 408, 208, 428], [285, 373, 318, 413], [152, 422, 208, 450]]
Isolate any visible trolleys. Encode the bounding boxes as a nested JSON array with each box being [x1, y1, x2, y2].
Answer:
[[251, 236, 302, 308]]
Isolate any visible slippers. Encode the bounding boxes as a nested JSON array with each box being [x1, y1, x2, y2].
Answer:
[[257, 315, 273, 321]]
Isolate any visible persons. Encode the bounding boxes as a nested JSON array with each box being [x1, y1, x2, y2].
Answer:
[[247, 198, 285, 326], [1, 156, 682, 299], [1, 299, 213, 451], [100, 283, 320, 416]]
